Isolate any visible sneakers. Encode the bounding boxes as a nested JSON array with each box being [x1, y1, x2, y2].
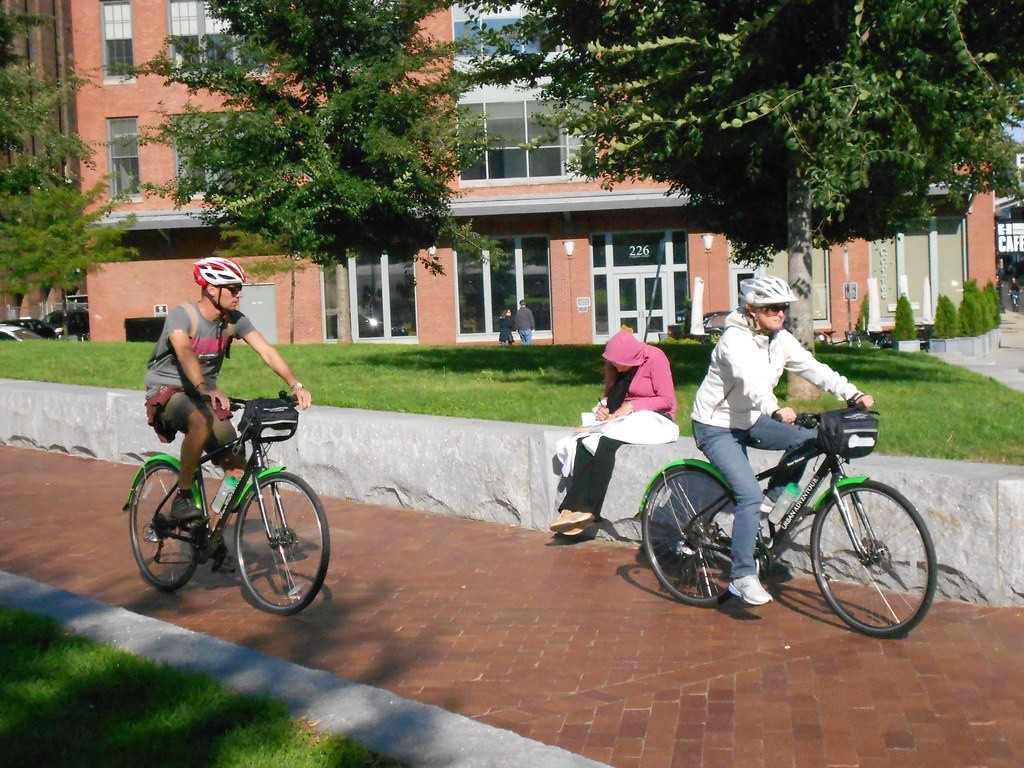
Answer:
[[207, 538, 236, 569], [729, 576, 773, 605], [761, 495, 814, 515], [170, 497, 202, 519]]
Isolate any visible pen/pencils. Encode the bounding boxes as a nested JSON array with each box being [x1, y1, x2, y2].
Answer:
[[598, 398, 604, 408]]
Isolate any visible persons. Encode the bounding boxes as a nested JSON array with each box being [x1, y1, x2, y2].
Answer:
[[515, 300, 535, 346], [498, 308, 516, 347], [996, 274, 1006, 313], [143, 254, 313, 575], [690, 277, 874, 604], [1008, 277, 1022, 303], [550, 328, 677, 535]]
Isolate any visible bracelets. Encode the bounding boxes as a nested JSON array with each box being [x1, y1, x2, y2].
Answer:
[[628, 401, 634, 412], [194, 382, 209, 389]]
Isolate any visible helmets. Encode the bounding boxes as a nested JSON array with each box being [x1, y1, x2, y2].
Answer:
[[193, 257, 246, 286], [740, 276, 799, 304]]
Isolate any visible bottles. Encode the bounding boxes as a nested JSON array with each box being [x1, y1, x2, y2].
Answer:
[[212, 476, 238, 513], [768, 482, 801, 524]]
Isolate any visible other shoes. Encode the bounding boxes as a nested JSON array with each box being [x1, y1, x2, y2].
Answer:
[[562, 522, 597, 535], [549, 511, 593, 531]]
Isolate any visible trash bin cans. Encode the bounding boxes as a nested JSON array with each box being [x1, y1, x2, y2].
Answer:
[[124, 316, 166, 342], [668, 325, 685, 340]]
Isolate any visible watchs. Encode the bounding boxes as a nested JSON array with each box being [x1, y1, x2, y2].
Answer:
[[290, 382, 305, 389]]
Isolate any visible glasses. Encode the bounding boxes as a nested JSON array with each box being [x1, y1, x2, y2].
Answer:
[[213, 284, 242, 293], [751, 304, 790, 316]]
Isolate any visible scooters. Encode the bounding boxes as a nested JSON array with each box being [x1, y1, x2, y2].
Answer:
[[1007, 290, 1023, 312]]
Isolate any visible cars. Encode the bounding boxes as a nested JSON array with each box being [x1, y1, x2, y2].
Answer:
[[0, 311, 90, 342], [667, 310, 732, 343]]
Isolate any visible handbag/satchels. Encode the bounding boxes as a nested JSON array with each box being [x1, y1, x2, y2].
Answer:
[[509, 331, 514, 343]]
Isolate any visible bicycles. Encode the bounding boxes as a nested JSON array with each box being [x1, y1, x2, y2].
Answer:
[[121, 389, 331, 616], [814, 328, 893, 349], [638, 392, 938, 640]]
